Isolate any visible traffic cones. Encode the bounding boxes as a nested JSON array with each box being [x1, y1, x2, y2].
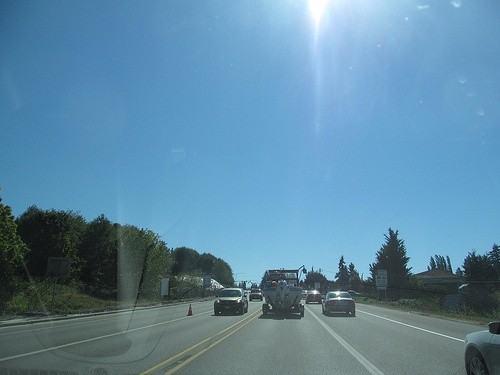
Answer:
[[187, 304, 193, 316]]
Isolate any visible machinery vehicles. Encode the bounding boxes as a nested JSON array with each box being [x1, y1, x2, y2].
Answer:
[[261, 269, 304, 318]]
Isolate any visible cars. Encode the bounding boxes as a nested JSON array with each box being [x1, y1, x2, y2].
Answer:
[[214, 288, 248, 315], [464, 321, 500, 375], [305, 290, 322, 304], [302, 290, 308, 298], [244, 289, 263, 301], [321, 291, 356, 316]]
